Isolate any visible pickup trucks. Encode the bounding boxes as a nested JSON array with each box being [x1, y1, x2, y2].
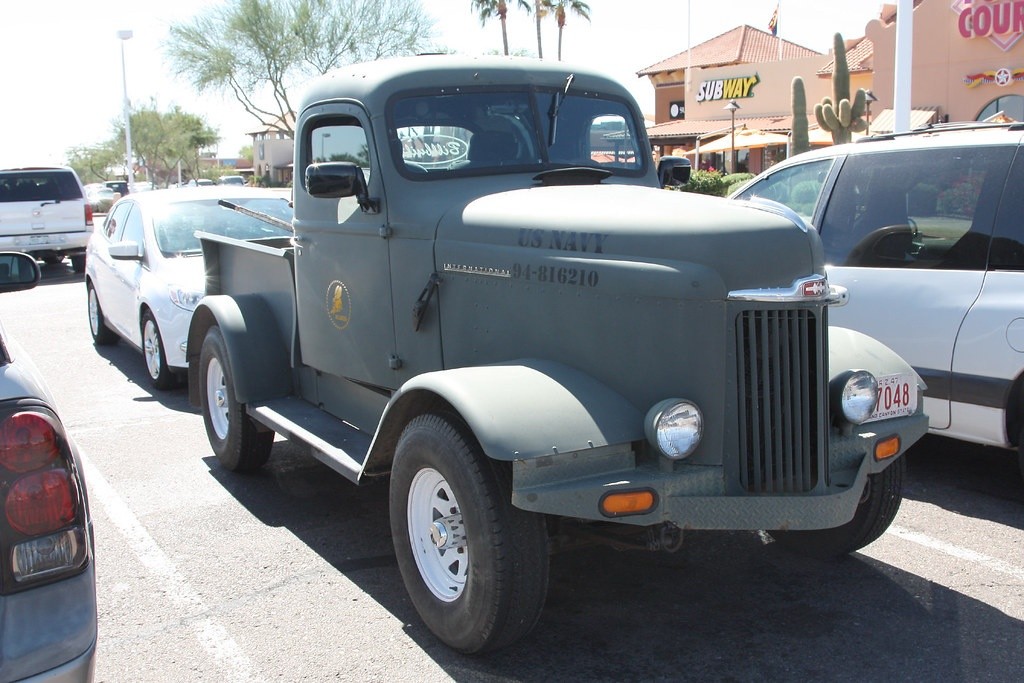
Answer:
[[182, 50, 925, 658]]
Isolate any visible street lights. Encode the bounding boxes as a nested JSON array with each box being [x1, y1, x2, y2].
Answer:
[[117, 29, 135, 188], [724, 101, 739, 174]]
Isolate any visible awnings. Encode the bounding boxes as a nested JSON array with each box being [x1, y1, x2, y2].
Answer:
[[869, 105, 939, 136]]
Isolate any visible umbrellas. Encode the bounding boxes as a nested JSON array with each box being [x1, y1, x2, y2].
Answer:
[[684, 123, 792, 156], [808, 125, 878, 144]]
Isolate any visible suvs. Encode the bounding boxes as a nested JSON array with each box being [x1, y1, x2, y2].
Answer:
[[0, 167, 95, 273]]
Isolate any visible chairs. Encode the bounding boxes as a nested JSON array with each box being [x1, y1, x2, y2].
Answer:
[[448, 131, 519, 170], [851, 188, 913, 257], [820, 193, 856, 265]]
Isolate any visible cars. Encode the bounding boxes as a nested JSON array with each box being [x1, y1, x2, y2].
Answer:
[[83, 180, 157, 212], [726, 120, 1024, 460], [0, 250, 98, 683], [85, 189, 296, 389], [214, 176, 247, 186], [169, 179, 216, 187]]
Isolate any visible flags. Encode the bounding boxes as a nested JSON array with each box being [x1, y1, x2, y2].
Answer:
[[768, 2, 779, 36]]
